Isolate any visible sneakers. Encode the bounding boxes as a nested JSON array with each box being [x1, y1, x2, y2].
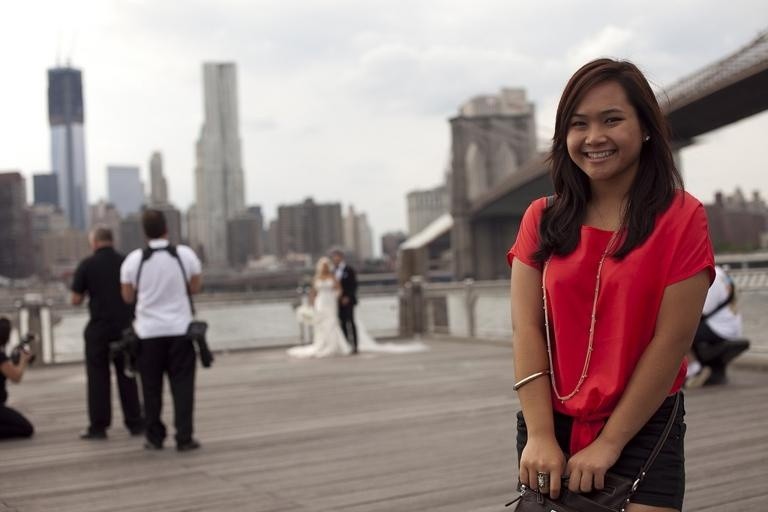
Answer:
[[79, 419, 202, 452]]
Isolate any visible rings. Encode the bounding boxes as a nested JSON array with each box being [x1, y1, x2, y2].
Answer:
[[536, 472, 548, 489]]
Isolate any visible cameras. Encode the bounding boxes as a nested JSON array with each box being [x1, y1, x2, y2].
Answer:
[[11, 332, 36, 365]]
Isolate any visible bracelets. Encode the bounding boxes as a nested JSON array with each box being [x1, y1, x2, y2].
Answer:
[[513, 369, 551, 391]]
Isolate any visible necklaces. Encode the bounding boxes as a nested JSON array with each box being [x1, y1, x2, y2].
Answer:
[[541, 222, 622, 403]]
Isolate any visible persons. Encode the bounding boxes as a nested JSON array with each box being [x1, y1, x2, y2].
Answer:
[[0, 315, 34, 443], [311, 248, 358, 353], [694, 264, 746, 395], [121, 211, 204, 451], [69, 225, 145, 440], [509, 58, 714, 512]]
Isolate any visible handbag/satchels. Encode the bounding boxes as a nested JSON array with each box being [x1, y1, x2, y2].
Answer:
[[511, 454, 636, 512]]
